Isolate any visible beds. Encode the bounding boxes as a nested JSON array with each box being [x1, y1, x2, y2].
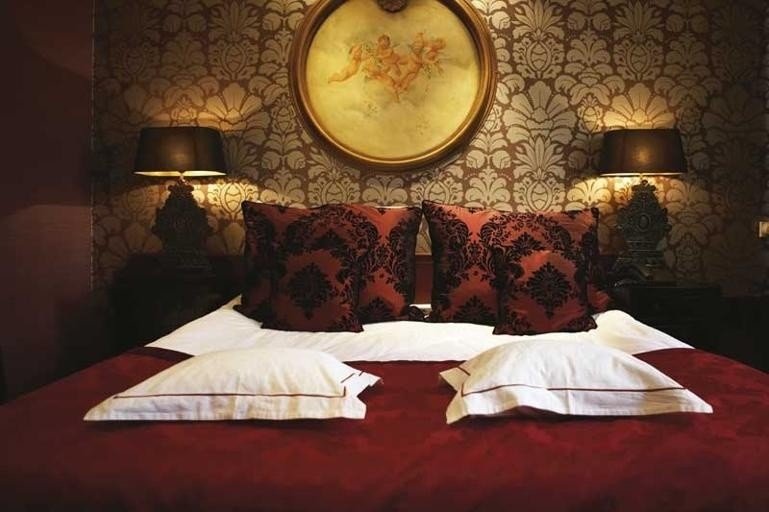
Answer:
[[0, 255, 768, 511]]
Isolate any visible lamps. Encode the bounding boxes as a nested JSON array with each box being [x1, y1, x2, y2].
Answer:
[[594, 128, 689, 285], [131, 125, 226, 270]]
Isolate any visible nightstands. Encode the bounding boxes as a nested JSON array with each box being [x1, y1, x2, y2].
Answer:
[[612, 282, 722, 353]]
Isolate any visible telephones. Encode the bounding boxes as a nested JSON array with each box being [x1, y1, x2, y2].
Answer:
[[630, 264, 677, 285]]
